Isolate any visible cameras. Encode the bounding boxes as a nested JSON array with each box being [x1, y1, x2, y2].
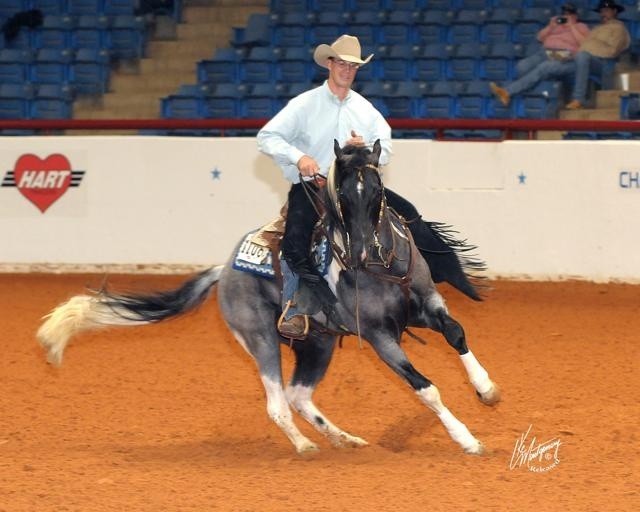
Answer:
[[556, 18, 566, 24]]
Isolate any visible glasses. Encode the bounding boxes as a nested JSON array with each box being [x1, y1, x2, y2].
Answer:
[[332, 59, 361, 69]]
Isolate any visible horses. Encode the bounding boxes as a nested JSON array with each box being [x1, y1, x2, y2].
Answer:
[[35, 139, 501, 458]]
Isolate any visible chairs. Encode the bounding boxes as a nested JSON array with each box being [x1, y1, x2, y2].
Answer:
[[0, 1, 640, 139]]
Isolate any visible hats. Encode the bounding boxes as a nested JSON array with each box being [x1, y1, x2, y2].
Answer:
[[591, 0, 625, 14], [561, 3, 578, 13], [312, 34, 374, 70]]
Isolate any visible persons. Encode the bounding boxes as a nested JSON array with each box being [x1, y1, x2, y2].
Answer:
[[489, 5, 630, 109], [256, 35, 443, 342], [512, 4, 590, 77]]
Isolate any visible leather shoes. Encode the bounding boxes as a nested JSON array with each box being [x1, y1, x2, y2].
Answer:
[[280, 315, 310, 340], [564, 101, 581, 110], [489, 82, 510, 107]]
